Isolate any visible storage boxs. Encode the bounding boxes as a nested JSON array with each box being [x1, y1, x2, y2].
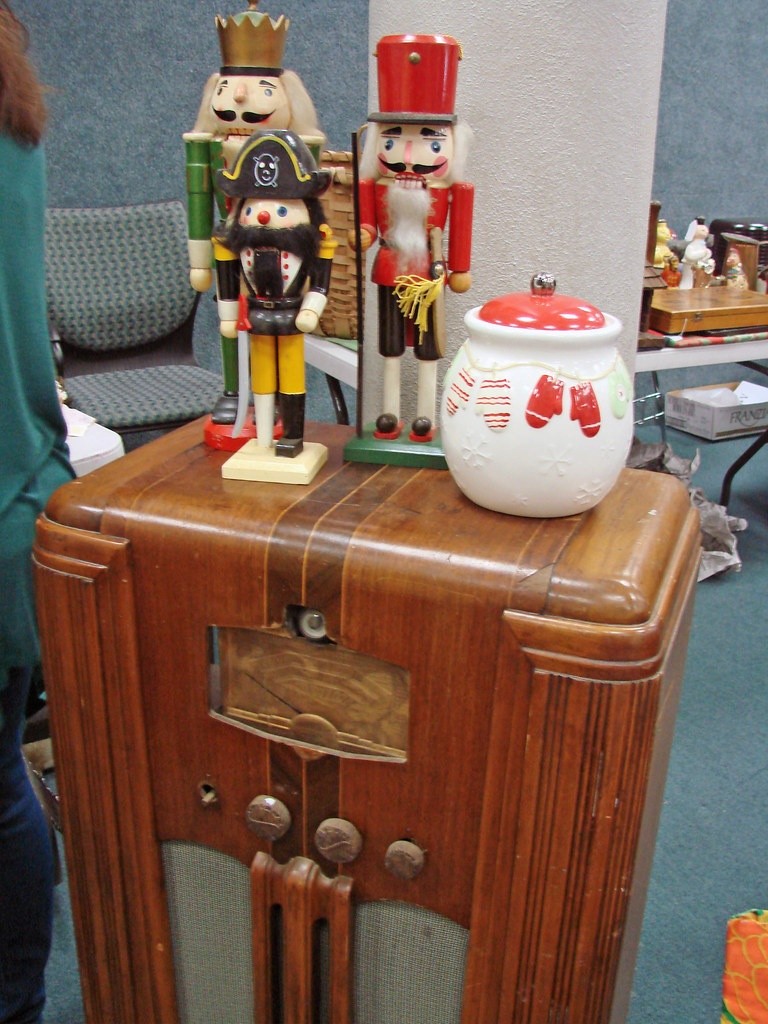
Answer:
[[662, 380, 768, 442]]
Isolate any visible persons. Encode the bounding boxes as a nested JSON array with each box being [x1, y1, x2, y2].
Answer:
[[348, 34, 474, 444], [653, 219, 674, 267], [182, 0, 325, 424], [210, 128, 338, 458], [0, 0, 78, 1024], [680, 216, 712, 289]]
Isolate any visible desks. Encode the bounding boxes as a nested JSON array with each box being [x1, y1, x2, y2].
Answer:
[[307, 333, 768, 505], [34, 408, 702, 1024]]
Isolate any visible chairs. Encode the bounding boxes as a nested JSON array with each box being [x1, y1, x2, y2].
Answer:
[[43, 199, 225, 455]]
